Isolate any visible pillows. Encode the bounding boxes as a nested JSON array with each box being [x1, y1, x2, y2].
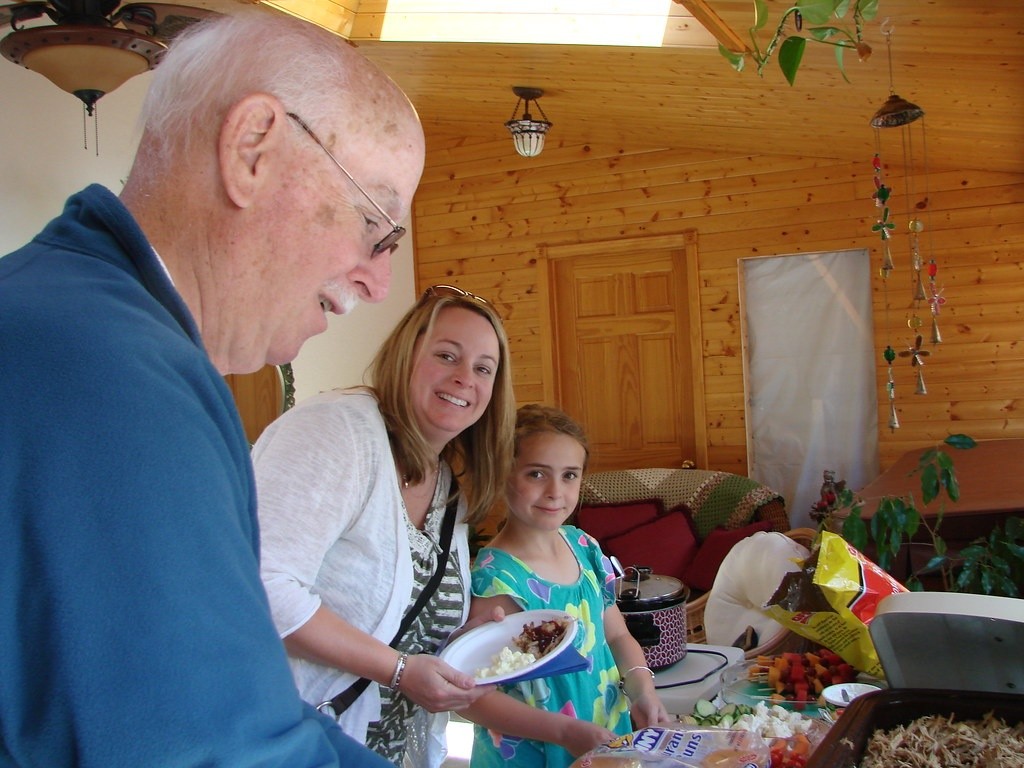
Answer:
[[604, 513, 697, 578], [577, 503, 656, 546], [703, 531, 810, 653], [681, 522, 771, 594]]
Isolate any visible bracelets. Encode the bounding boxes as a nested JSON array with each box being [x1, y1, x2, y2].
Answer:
[[390, 652, 407, 690], [619, 667, 655, 696]]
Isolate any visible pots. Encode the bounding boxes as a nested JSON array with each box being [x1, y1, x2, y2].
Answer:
[[616, 566, 691, 668]]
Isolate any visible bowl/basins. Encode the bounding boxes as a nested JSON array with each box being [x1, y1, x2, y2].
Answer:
[[821, 682, 882, 721]]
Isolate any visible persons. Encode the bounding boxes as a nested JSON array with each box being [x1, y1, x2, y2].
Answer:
[[470, 403, 670, 768], [0, 12, 424, 768], [248, 284, 505, 768]]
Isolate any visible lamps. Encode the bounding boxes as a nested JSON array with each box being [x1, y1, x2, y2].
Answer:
[[0, 0, 231, 159], [504, 86, 551, 158]]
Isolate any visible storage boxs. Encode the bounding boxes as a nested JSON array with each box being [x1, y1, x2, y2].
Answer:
[[805, 690, 1024, 768]]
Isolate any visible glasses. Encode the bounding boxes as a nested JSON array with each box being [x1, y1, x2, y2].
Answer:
[[424, 283, 504, 324], [286, 111, 408, 259]]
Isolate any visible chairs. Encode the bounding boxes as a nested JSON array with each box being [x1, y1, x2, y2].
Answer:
[[562, 469, 790, 533], [687, 528, 821, 659]]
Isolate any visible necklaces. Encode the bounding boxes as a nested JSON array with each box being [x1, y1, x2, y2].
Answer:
[[403, 460, 438, 488]]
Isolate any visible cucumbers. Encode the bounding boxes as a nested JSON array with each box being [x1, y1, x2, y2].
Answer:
[[693, 699, 751, 726]]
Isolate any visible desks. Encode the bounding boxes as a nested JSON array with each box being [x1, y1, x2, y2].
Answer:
[[628, 642, 745, 735], [831, 438, 1024, 593]]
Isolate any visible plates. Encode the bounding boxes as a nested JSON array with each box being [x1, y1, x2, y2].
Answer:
[[438, 609, 577, 685]]
